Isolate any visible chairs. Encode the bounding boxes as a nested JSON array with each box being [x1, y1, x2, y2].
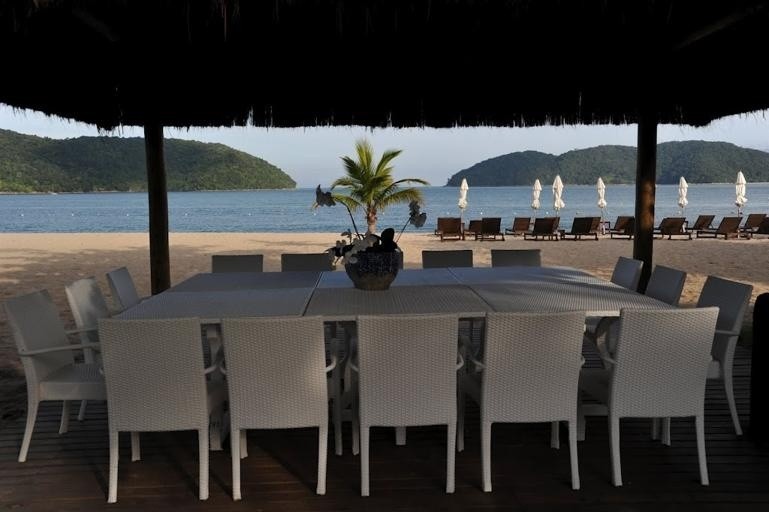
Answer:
[[422, 250, 473, 266], [468, 310, 586, 493], [220, 315, 343, 501], [281, 253, 335, 272], [97, 317, 224, 504], [595, 306, 721, 488], [5, 268, 154, 464], [610, 255, 755, 437], [212, 255, 263, 272], [346, 314, 465, 497], [492, 249, 540, 266]]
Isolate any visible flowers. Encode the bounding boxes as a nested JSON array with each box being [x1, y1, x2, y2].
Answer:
[[315, 183, 426, 265]]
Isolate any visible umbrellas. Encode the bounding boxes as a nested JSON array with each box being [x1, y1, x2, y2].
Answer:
[[552, 175, 565, 217], [597, 177, 607, 221], [734, 170, 748, 217], [458, 177, 469, 222], [532, 179, 542, 217], [678, 176, 688, 217]]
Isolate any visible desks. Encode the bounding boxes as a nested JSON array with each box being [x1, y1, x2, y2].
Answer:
[[110, 266, 681, 463]]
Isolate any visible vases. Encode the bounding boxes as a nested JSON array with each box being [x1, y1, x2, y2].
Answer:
[[345, 248, 401, 291]]
